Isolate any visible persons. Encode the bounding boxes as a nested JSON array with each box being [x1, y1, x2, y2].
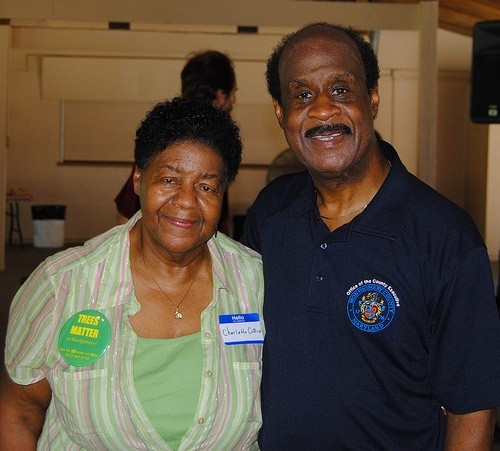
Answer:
[[114, 50, 239, 241], [0, 96, 267, 451], [237, 21, 500, 451]]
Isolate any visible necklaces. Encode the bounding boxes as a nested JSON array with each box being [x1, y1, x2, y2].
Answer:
[[315, 159, 391, 220], [140, 243, 204, 319]]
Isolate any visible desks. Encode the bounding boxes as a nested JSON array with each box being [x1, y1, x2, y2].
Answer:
[[6, 194, 33, 247]]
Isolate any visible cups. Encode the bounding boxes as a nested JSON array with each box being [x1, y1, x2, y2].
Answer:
[[30, 204, 67, 248]]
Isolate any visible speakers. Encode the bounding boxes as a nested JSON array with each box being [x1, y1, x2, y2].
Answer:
[[470, 19, 500, 124]]
